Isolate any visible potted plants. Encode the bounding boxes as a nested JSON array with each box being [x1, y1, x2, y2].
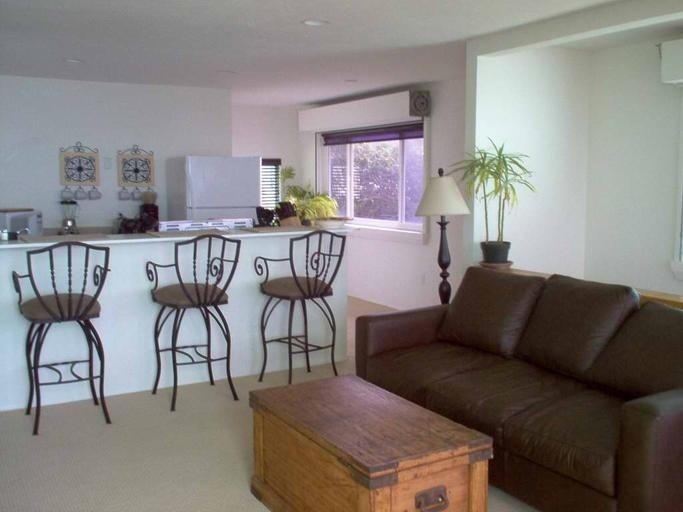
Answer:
[[290, 183, 339, 227], [444, 138, 534, 264]]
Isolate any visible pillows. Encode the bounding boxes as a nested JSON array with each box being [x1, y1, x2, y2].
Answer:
[[436, 267, 544, 361], [585, 299, 682, 398], [516, 273, 636, 377]]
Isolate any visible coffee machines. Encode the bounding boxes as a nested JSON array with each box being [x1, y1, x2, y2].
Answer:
[[138, 192, 159, 233]]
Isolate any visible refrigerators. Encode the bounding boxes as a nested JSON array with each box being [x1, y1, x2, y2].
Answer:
[[165, 156, 261, 225]]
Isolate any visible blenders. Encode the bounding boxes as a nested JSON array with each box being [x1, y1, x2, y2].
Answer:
[[58, 200, 78, 234]]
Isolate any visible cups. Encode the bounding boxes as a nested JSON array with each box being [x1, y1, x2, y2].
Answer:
[[0, 232, 7, 241], [87, 187, 101, 200], [131, 188, 142, 200], [61, 188, 73, 200], [74, 186, 88, 200], [118, 188, 130, 201]]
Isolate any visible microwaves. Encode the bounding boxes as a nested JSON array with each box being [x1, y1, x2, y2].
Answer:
[[0, 211, 43, 235]]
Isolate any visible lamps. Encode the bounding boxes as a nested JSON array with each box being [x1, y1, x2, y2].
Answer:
[[413, 167, 474, 303]]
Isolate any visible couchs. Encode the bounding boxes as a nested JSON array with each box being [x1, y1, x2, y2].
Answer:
[[356, 264, 682, 511]]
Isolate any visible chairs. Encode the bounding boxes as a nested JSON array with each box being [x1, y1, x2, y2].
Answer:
[[146, 233, 239, 411], [11, 241, 112, 436], [255, 229, 343, 384]]
